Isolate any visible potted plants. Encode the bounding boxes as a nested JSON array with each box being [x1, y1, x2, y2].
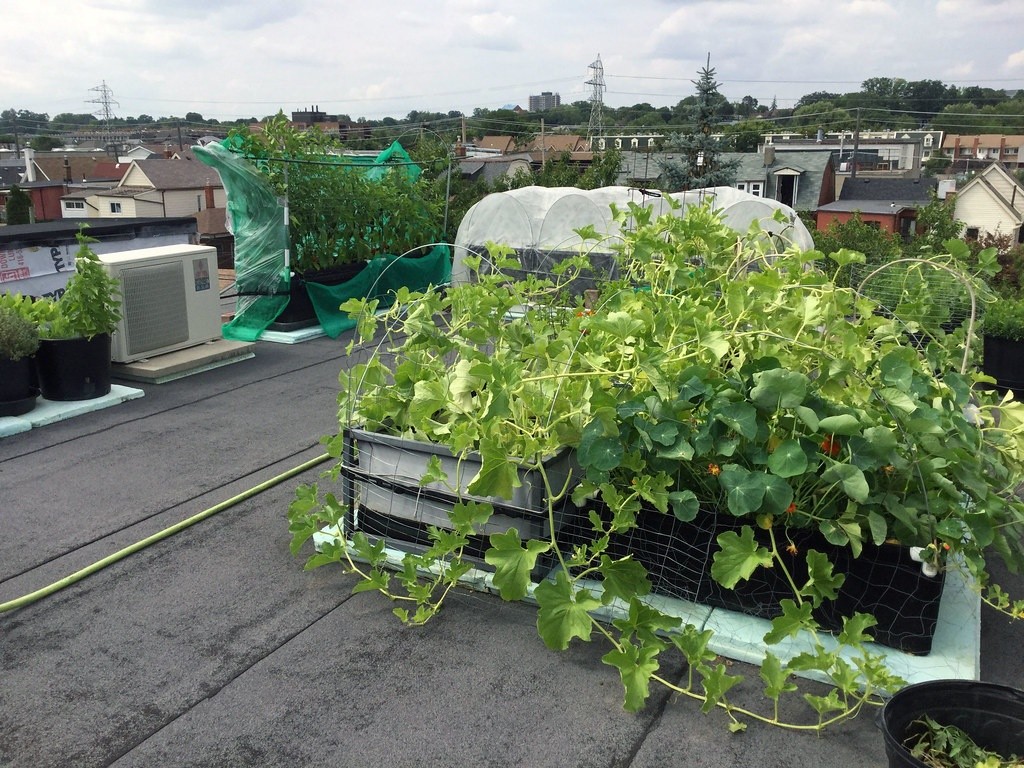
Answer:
[[286, 191, 1024, 768], [0, 221, 125, 418]]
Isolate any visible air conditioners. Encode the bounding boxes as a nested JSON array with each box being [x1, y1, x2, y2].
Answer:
[[75, 245, 221, 364]]
